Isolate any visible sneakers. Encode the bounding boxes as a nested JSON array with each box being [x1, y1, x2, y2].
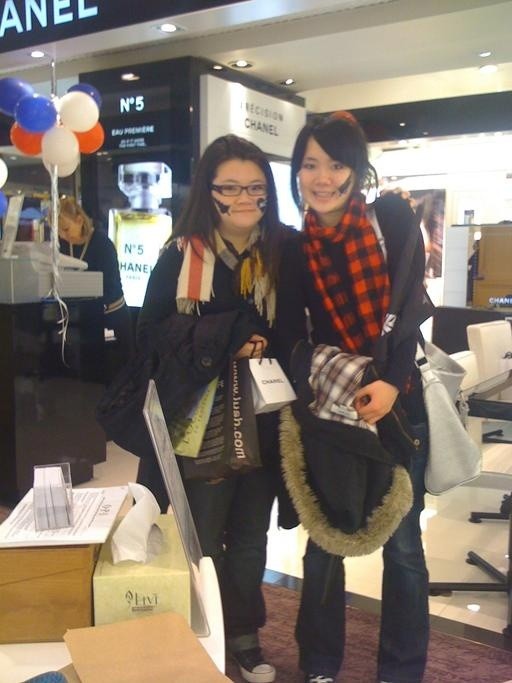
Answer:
[[231, 648, 276, 683]]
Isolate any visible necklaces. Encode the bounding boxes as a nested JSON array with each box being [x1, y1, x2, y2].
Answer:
[[67, 240, 90, 260]]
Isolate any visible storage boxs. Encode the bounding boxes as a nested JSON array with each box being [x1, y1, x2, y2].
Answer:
[[0, 488, 102, 646]]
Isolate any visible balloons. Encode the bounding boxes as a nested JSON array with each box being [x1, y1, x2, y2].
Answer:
[[0, 76, 106, 219]]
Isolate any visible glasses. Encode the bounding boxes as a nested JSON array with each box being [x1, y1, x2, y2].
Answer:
[[210, 183, 267, 196]]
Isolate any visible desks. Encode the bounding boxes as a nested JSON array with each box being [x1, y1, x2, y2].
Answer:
[[456, 371, 512, 513]]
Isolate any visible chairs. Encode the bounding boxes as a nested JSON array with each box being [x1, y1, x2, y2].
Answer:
[[419, 351, 511, 524], [465, 318, 511, 424]]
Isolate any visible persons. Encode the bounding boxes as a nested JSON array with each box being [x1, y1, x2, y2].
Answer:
[[46, 194, 131, 351], [131, 133, 300, 682], [467, 240, 479, 303], [279, 110, 431, 683]]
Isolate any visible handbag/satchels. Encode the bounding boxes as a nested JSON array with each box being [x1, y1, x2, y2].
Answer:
[[167, 341, 299, 480], [419, 340, 482, 495]]
